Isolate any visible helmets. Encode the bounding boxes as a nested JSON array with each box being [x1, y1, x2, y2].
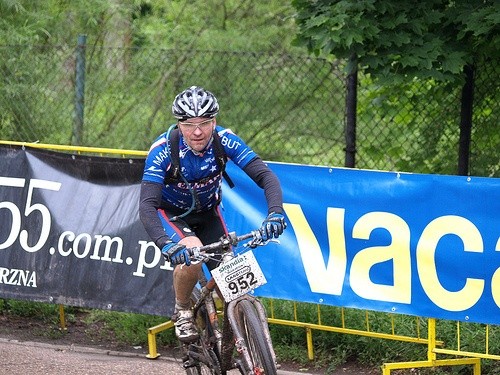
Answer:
[[171, 85, 221, 121]]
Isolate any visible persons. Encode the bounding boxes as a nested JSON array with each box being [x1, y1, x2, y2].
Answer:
[[139, 87, 285, 365]]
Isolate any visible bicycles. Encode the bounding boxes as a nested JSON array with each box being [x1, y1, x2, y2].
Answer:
[[163, 222, 278, 375]]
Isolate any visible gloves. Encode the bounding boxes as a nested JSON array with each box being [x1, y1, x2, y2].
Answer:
[[161, 242, 192, 267], [260, 211, 289, 241]]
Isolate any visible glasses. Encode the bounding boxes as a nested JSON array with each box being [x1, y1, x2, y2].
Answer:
[[178, 119, 215, 133]]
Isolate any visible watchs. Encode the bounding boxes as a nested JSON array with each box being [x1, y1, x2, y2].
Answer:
[[161, 240, 176, 251]]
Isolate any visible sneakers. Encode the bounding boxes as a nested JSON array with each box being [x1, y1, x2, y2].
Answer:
[[171, 304, 201, 343]]
